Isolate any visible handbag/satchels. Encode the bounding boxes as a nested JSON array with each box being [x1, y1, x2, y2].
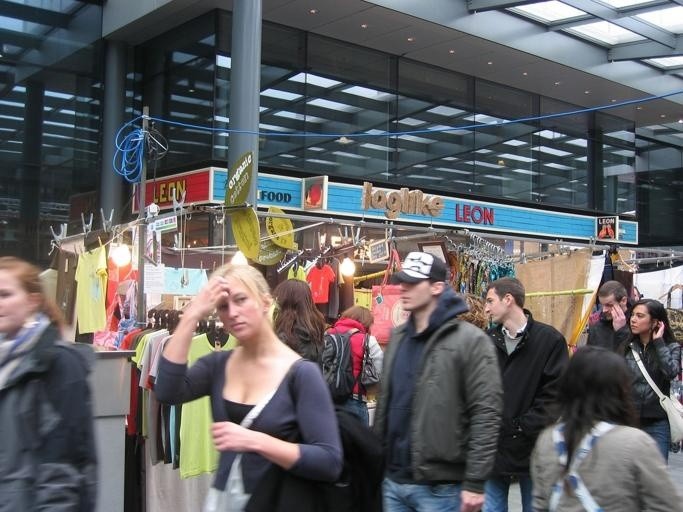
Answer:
[[362, 356, 382, 393], [660, 395, 683, 442], [217, 455, 252, 511]]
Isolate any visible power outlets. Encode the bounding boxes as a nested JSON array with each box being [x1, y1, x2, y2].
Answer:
[[147, 203, 158, 217]]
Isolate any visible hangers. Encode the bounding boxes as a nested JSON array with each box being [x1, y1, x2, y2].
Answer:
[[466, 233, 506, 261], [148, 309, 229, 348]]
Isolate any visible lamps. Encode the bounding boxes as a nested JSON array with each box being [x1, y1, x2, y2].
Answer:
[[231, 248, 249, 267], [338, 254, 356, 278], [110, 239, 132, 268]]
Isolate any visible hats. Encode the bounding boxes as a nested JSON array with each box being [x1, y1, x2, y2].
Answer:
[[392, 252, 446, 284]]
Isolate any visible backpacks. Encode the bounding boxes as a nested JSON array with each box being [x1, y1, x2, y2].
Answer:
[[321, 328, 360, 405]]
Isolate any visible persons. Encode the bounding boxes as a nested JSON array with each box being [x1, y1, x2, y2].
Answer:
[[625, 298, 682, 464], [480, 276, 570, 512], [267, 279, 325, 359], [323, 305, 385, 425], [587, 279, 631, 352], [0, 255, 98, 512], [528, 344, 683, 512], [371, 251, 506, 512], [151, 261, 347, 512]]
